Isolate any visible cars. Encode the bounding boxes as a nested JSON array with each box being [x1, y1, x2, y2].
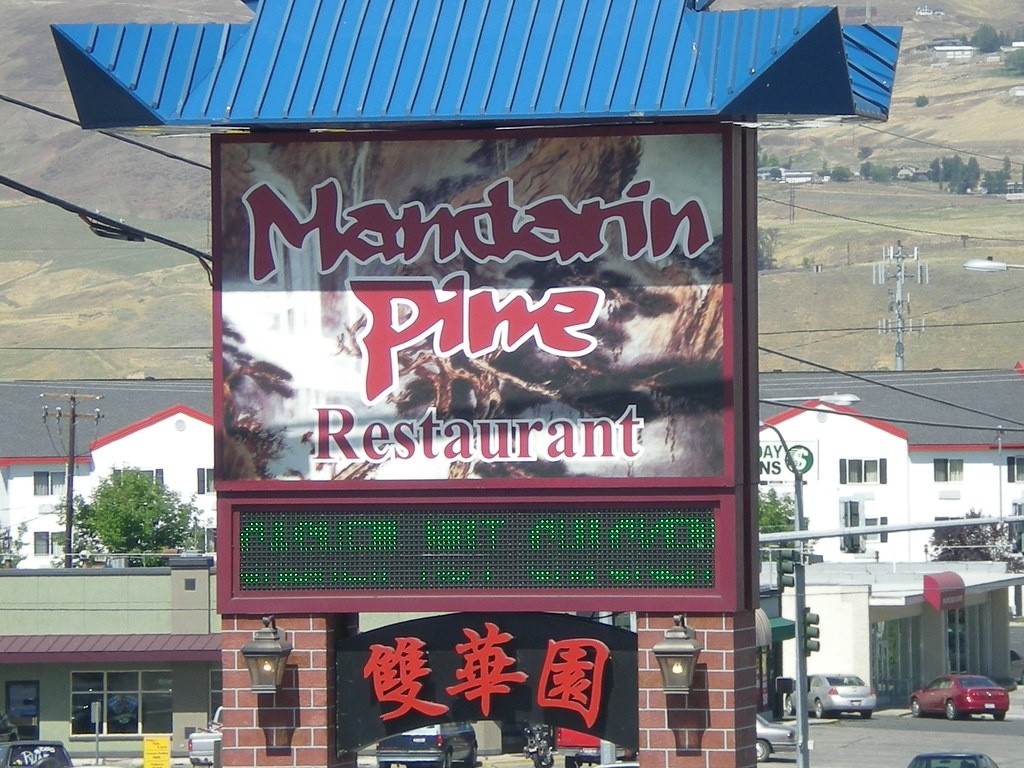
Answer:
[[755, 711, 796, 762], [907, 753, 999, 768], [909, 673, 1010, 721], [376, 720, 478, 768], [555, 726, 637, 768], [785, 673, 877, 719]]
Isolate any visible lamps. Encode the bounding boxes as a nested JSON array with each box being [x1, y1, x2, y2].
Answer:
[[240, 614, 292, 694], [653, 614, 704, 695]]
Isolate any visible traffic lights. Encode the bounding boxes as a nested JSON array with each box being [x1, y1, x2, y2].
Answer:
[[803, 606, 819, 656], [777, 547, 795, 595]]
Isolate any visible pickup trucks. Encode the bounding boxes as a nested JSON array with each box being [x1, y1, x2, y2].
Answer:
[[189, 705, 223, 766]]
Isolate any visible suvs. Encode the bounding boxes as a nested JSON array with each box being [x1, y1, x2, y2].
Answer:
[[0, 739, 74, 768]]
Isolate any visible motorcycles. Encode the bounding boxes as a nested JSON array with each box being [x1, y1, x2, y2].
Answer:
[[523, 726, 558, 768]]
